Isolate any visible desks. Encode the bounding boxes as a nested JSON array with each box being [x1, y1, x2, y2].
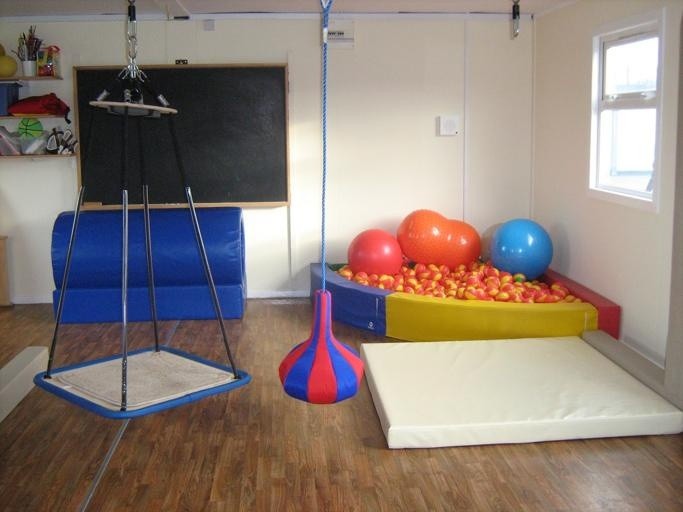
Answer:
[[33, 185, 252, 420]]
